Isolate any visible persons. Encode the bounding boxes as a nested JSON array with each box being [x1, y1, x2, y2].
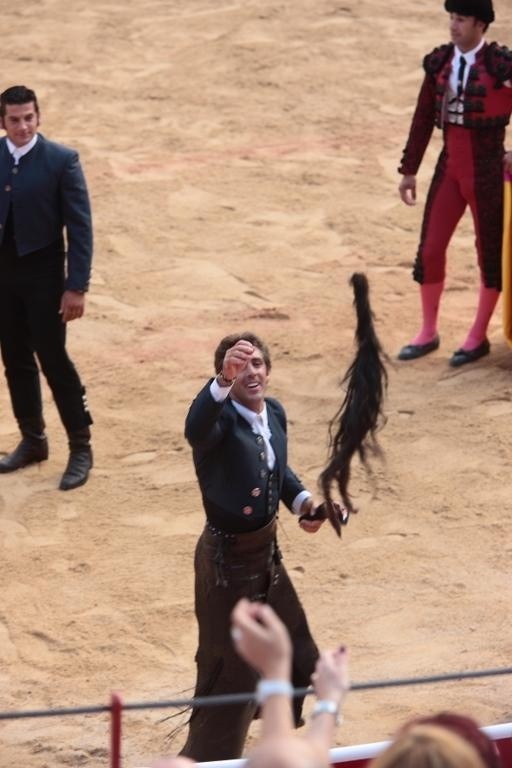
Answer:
[[395, 1, 511, 367], [173, 331, 350, 768], [229, 597, 490, 768], [0, 84, 93, 490], [307, 643, 508, 768]]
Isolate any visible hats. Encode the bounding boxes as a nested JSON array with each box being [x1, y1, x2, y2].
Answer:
[[444, 1, 495, 23]]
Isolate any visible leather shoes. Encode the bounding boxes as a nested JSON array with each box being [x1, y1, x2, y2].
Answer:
[[399, 334, 440, 359], [449, 339, 490, 366]]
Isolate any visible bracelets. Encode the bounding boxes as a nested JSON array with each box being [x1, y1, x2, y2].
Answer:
[[254, 678, 295, 706], [310, 699, 344, 727]]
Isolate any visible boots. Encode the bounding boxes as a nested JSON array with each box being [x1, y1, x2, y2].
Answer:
[[0, 411, 48, 472], [59, 405, 92, 490]]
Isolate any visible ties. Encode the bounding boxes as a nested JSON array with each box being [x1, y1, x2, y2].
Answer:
[[457, 56, 466, 96]]
